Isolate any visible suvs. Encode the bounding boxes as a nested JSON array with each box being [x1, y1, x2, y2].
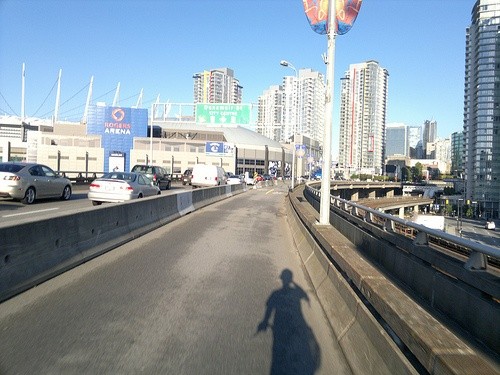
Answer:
[[132, 165, 171, 190]]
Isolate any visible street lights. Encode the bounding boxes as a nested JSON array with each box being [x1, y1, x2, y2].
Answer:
[[279, 60, 298, 188]]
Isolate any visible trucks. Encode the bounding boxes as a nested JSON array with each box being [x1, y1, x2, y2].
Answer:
[[486, 222, 496, 230]]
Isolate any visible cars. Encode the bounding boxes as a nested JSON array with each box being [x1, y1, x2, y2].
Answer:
[[180, 164, 271, 188], [0, 162, 72, 204], [87, 172, 162, 206]]
